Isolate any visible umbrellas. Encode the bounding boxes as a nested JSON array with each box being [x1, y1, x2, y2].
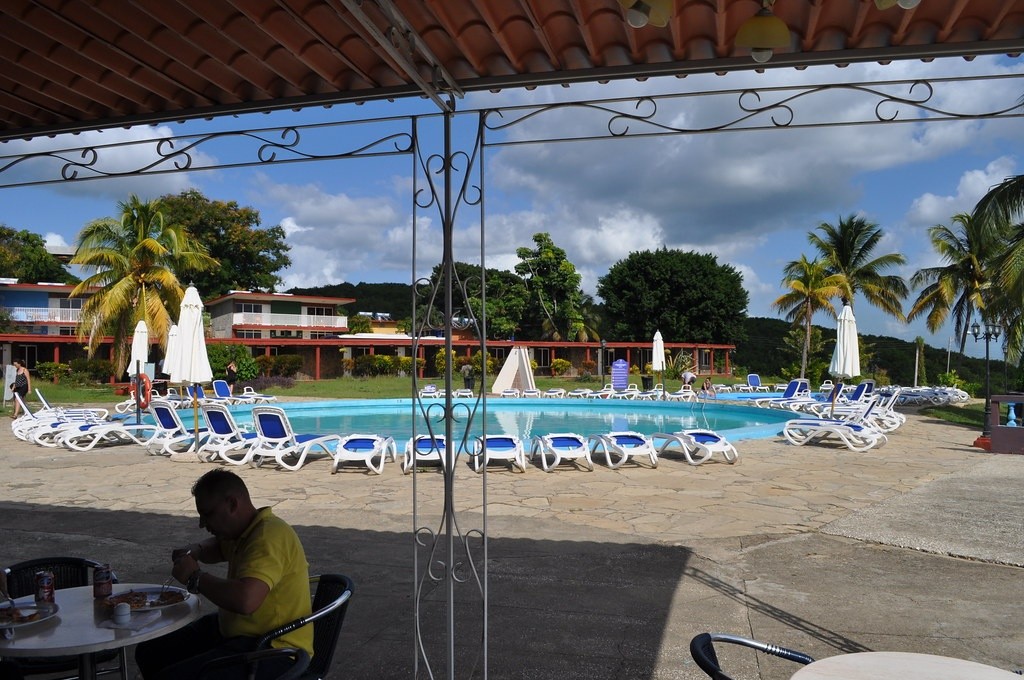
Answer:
[[126, 361, 145, 376], [827, 301, 861, 418], [162, 324, 184, 411], [651, 329, 666, 386], [131, 320, 148, 363], [169, 280, 213, 453]]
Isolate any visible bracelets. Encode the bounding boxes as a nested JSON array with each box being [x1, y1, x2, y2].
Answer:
[[187, 570, 208, 594]]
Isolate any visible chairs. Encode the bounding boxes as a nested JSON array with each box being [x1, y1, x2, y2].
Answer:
[[0, 556, 118, 600], [181, 648, 311, 679], [10, 379, 342, 472], [475, 435, 524, 474], [690, 632, 815, 680], [651, 429, 738, 467], [418, 374, 969, 453], [252, 573, 355, 680], [403, 434, 455, 473], [586, 431, 659, 469], [530, 432, 594, 472], [332, 434, 397, 474]]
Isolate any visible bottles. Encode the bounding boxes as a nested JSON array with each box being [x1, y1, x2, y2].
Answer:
[[114, 602, 130, 624]]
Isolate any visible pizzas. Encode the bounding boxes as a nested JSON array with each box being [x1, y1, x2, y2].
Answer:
[[103, 589, 184, 607], [0, 606, 40, 625]]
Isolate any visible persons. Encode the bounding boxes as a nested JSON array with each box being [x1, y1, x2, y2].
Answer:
[[134, 469, 315, 680], [701, 377, 715, 398], [10, 359, 32, 419], [226, 361, 237, 396]]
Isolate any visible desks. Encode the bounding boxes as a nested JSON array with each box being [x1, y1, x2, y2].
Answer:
[[789, 652, 1024, 679]]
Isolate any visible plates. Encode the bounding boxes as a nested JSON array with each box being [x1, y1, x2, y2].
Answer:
[[0, 602, 59, 628], [106, 586, 191, 611]]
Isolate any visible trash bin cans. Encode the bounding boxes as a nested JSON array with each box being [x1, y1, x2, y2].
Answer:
[[641, 376, 653, 392], [464, 377, 475, 389]]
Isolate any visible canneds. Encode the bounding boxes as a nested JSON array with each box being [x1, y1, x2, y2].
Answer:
[[34, 570, 57, 603], [93, 562, 112, 598]]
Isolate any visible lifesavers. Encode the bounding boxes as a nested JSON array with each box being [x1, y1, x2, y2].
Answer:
[[132, 373, 151, 409]]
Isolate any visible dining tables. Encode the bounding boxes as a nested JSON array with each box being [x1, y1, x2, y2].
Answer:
[[0, 583, 201, 680]]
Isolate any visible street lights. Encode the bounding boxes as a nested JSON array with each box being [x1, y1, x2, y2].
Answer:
[[971, 319, 1002, 450], [602, 338, 606, 387], [947, 331, 972, 376]]
